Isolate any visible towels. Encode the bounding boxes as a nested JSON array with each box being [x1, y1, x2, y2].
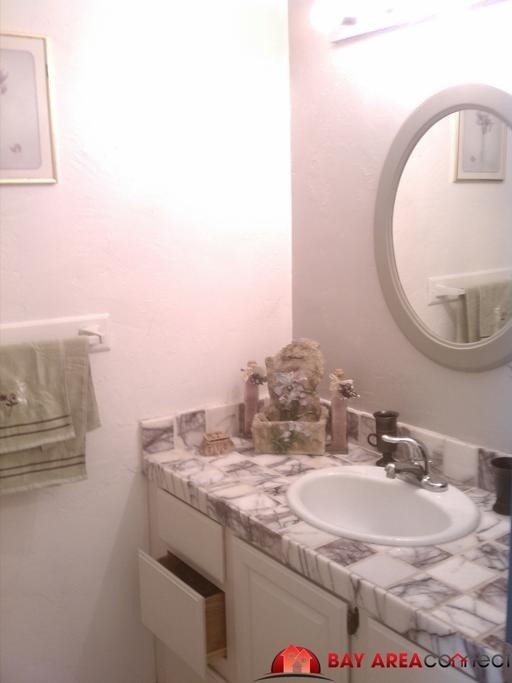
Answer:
[[457, 278, 511, 344], [0, 337, 102, 496]]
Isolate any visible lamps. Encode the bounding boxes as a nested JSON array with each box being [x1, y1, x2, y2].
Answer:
[[308, 1, 487, 44]]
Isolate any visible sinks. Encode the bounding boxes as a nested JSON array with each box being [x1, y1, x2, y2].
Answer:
[[286, 464, 481, 548]]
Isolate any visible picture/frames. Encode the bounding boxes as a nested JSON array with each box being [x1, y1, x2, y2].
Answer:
[[0, 32, 60, 185], [453, 109, 507, 182]]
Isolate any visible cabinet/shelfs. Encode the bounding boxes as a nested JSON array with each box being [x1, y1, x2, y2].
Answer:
[[137, 479, 484, 683]]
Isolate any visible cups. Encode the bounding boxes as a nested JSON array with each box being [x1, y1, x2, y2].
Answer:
[[365, 408, 400, 465], [487, 456, 512, 516]]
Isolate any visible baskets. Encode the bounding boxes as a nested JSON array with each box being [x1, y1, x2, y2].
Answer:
[[201, 434, 235, 457]]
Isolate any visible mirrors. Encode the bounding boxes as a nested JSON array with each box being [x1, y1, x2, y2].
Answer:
[[371, 77, 511, 373]]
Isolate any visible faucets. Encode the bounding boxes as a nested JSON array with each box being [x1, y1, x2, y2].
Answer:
[[381, 433, 449, 491]]
